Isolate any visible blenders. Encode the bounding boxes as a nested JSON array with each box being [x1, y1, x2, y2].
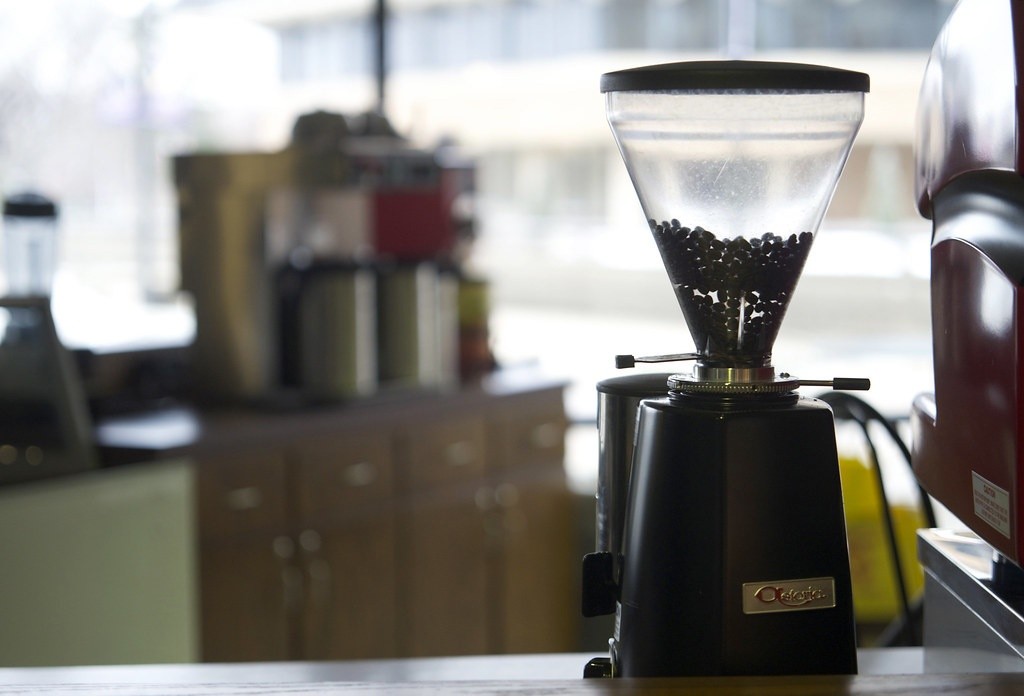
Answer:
[[589, 61, 872, 670], [0, 195, 96, 472]]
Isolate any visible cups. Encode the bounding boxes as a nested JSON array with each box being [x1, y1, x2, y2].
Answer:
[[381, 257, 460, 391], [274, 260, 379, 401]]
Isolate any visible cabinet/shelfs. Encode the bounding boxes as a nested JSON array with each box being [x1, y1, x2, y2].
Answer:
[[198, 391, 586, 663]]
